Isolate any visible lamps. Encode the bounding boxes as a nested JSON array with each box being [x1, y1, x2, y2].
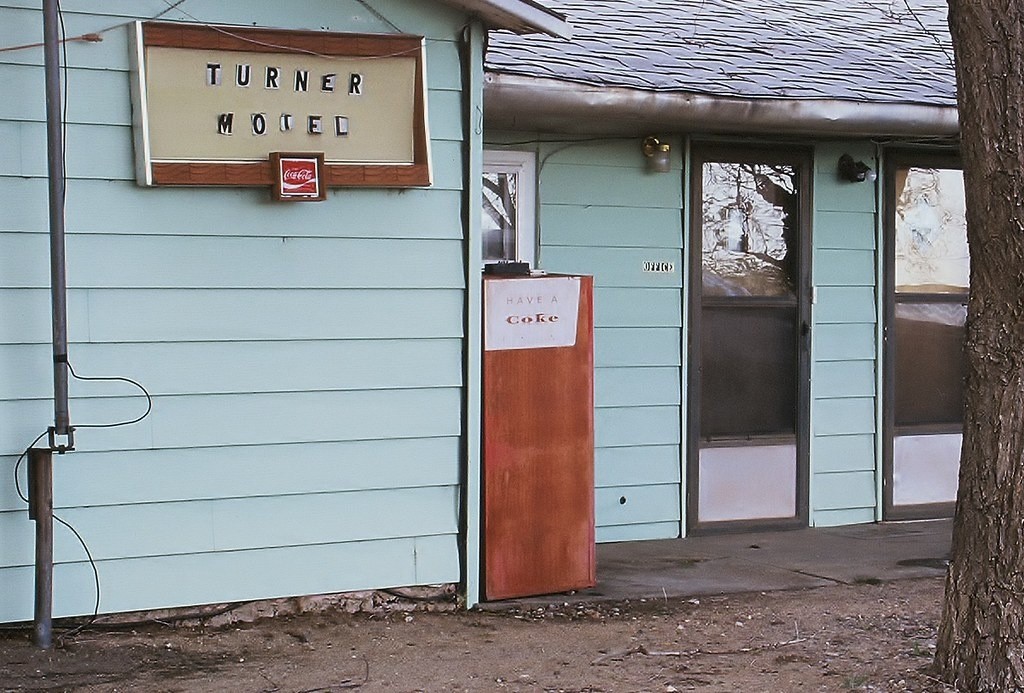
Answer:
[[838, 154, 875, 185], [642, 136, 672, 174]]
